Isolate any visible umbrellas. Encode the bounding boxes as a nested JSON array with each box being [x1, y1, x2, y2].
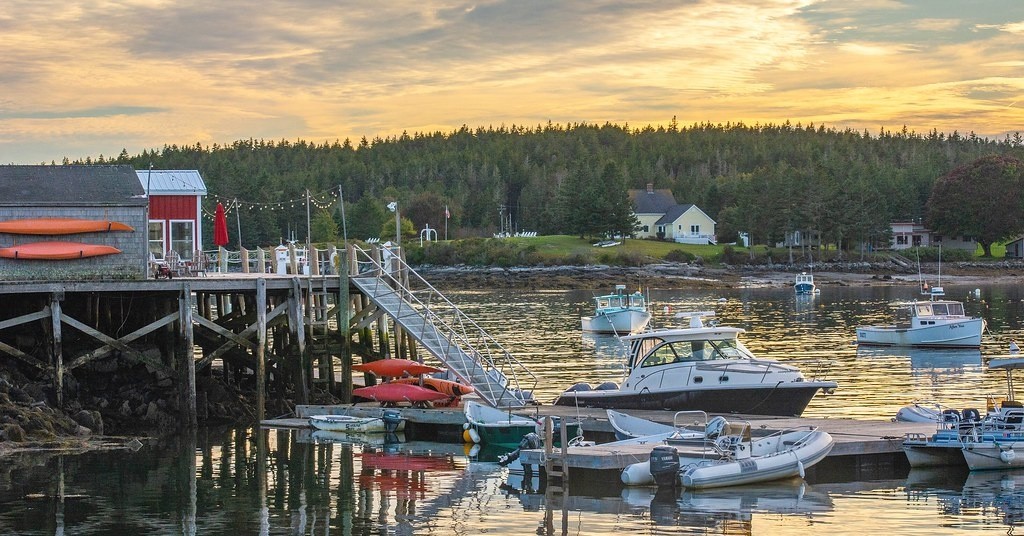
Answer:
[[214, 203, 228, 276]]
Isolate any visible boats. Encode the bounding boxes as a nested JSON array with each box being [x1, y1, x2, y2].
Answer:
[[794, 272, 820, 293], [349, 359, 474, 402], [497, 409, 705, 477], [621, 416, 836, 489], [855, 244, 987, 348], [308, 414, 410, 433], [552, 286, 838, 418], [312, 430, 405, 449], [901, 408, 1024, 471], [907, 467, 1024, 529], [464, 401, 582, 452], [581, 285, 652, 333], [896, 358, 1024, 422], [857, 344, 983, 380], [620, 477, 834, 536]]
